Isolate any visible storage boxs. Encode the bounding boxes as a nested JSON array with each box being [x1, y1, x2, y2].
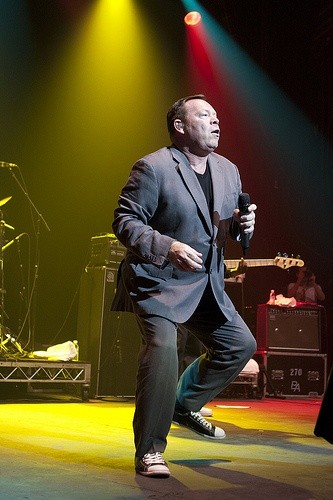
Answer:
[[256, 351, 327, 397]]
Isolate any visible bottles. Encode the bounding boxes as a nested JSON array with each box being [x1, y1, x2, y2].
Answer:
[[72, 340, 79, 361], [269, 289, 275, 304]]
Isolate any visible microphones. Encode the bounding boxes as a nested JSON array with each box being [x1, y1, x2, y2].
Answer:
[[238, 193, 251, 257]]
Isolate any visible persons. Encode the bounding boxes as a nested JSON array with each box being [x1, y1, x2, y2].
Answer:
[[288, 267, 325, 303], [112, 95, 256, 476]]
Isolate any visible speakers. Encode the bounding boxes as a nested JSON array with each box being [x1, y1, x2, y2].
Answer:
[[256, 304, 322, 352], [78, 267, 142, 399]]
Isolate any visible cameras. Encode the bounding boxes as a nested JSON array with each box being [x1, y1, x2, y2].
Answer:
[[302, 269, 312, 278]]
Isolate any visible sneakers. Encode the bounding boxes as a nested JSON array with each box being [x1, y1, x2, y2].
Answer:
[[135, 452, 171, 476], [172, 410, 226, 439]]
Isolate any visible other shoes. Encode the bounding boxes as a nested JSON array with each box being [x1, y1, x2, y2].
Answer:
[[200, 408, 212, 415]]
